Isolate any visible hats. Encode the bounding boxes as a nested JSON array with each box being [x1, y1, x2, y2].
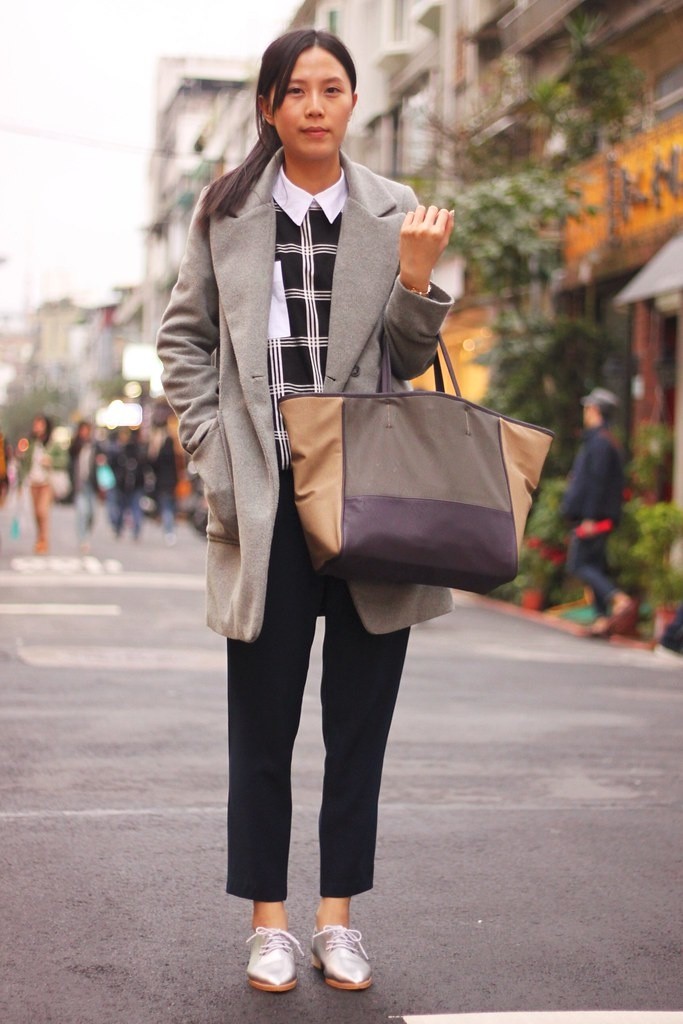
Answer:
[[580, 387, 618, 415]]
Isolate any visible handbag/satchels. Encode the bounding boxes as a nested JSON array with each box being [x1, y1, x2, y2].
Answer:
[[278, 334, 555, 595], [97, 464, 117, 488], [51, 469, 73, 497]]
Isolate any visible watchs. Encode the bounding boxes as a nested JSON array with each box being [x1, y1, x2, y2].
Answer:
[[408, 284, 431, 298]]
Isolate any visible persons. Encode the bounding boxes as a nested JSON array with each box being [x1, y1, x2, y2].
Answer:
[[557, 386, 636, 636], [0, 414, 70, 554], [155, 29, 456, 989], [148, 437, 177, 534], [69, 421, 159, 539]]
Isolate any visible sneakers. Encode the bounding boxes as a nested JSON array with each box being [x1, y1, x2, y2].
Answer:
[[245, 927, 306, 992], [311, 924, 373, 990]]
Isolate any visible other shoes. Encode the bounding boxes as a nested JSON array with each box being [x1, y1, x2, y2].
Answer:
[[585, 626, 613, 644]]
